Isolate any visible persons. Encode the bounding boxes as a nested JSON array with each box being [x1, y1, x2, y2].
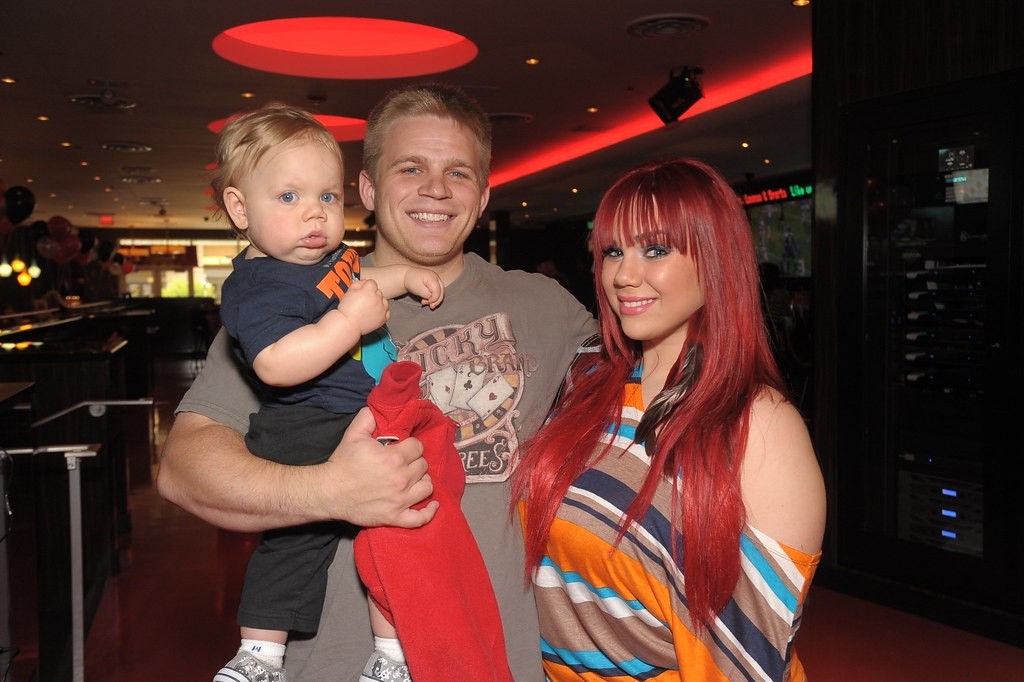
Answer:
[[503, 156, 827, 682], [202, 104, 412, 682], [154, 80, 604, 681]]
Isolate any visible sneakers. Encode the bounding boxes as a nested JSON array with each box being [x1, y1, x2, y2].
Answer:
[[358, 649, 413, 682], [212, 650, 287, 682]]
